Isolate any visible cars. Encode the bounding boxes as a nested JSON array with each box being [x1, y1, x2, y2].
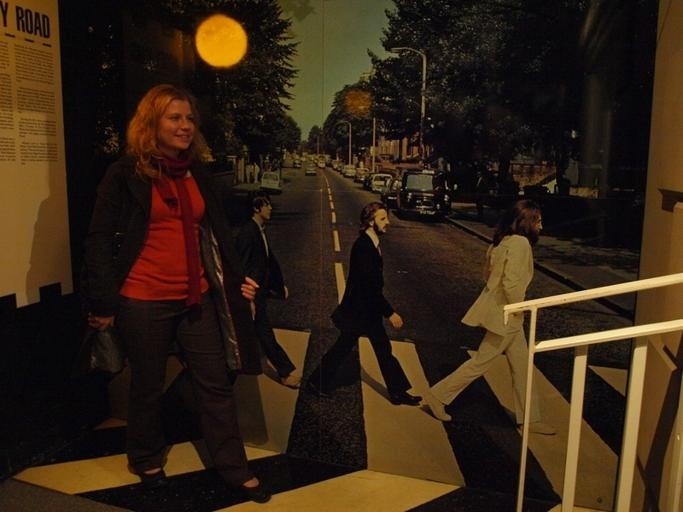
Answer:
[[257, 149, 451, 219]]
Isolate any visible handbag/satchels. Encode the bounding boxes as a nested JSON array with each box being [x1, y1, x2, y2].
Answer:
[[81, 311, 126, 381]]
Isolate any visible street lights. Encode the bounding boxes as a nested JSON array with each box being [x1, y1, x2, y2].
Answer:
[[338, 119, 351, 165], [391, 45, 426, 162]]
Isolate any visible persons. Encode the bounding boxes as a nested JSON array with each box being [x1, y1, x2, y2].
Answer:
[[424, 198, 558, 436], [234, 192, 305, 387], [86, 82, 272, 504], [307, 201, 423, 407]]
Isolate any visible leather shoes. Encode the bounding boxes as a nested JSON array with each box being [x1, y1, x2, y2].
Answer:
[[282, 375, 302, 389], [519, 417, 555, 436], [306, 377, 334, 399], [233, 475, 271, 503], [137, 463, 165, 485], [418, 392, 453, 422], [392, 389, 422, 405]]
